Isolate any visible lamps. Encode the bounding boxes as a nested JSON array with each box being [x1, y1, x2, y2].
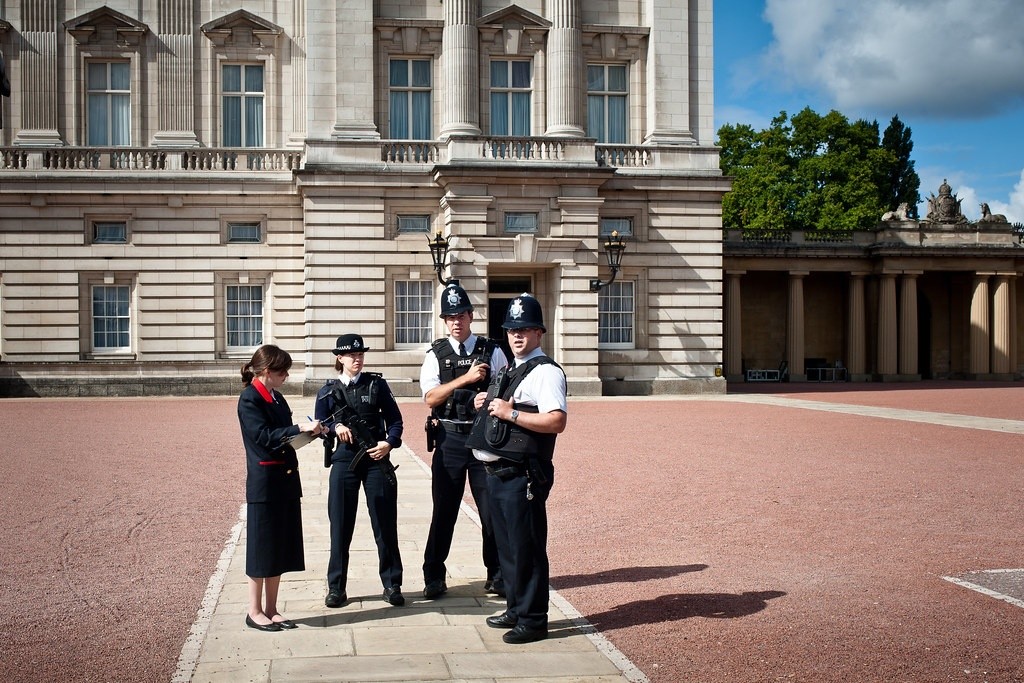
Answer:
[[590, 230, 626, 292], [424, 230, 459, 288]]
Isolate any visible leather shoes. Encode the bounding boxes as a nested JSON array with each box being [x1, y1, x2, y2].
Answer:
[[424, 581, 448, 599], [383, 584, 405, 605], [487, 613, 517, 627], [484, 581, 506, 597], [246, 613, 280, 631], [265, 614, 295, 628], [503, 625, 549, 643], [326, 590, 347, 608]]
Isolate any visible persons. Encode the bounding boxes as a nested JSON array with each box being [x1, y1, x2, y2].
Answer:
[[463, 292, 569, 645], [418, 286, 507, 600], [237, 344, 330, 633], [314, 333, 406, 609]]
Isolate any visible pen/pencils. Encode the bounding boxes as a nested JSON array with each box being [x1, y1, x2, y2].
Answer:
[[306, 414, 328, 437]]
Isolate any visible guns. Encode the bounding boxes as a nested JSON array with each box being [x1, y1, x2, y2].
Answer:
[[327, 389, 398, 488]]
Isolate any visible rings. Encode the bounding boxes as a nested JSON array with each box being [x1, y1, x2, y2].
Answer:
[[376, 454, 379, 457], [491, 406, 494, 410]]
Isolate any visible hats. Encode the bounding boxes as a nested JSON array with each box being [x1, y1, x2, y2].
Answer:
[[439, 287, 474, 318], [500, 297, 547, 333], [332, 334, 369, 354]]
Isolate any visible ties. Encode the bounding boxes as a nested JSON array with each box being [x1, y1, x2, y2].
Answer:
[[459, 344, 467, 357], [511, 359, 516, 370]]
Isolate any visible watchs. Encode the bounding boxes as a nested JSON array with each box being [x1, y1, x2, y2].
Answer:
[[511, 409, 519, 423]]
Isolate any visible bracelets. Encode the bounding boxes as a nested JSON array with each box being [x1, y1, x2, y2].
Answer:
[[335, 423, 343, 430]]
